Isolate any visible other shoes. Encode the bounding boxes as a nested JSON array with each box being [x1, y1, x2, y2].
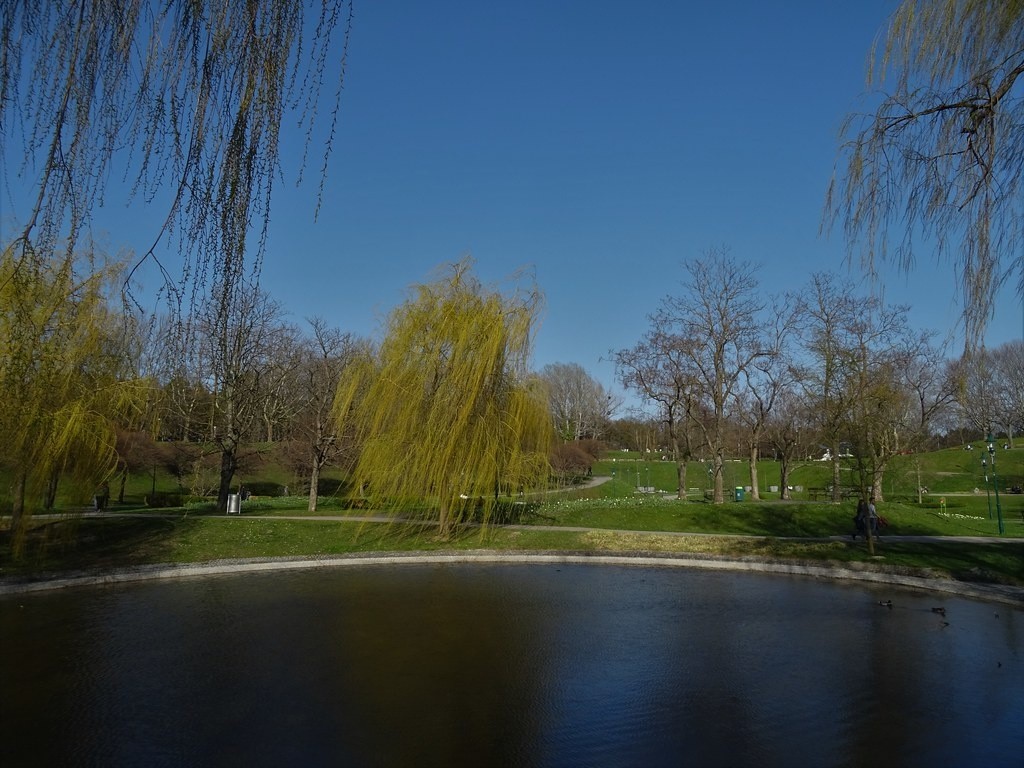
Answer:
[[852, 533, 856, 540]]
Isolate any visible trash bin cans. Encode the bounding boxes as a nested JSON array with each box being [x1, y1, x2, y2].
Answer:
[[735, 486, 743, 502], [807, 487, 818, 501]]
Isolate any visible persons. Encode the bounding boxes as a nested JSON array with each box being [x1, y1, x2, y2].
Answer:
[[852, 497, 882, 540], [919, 486, 928, 493], [967, 444, 970, 449], [1003, 442, 1008, 449], [588, 465, 592, 475], [103, 482, 111, 509], [1012, 485, 1021, 494]]
